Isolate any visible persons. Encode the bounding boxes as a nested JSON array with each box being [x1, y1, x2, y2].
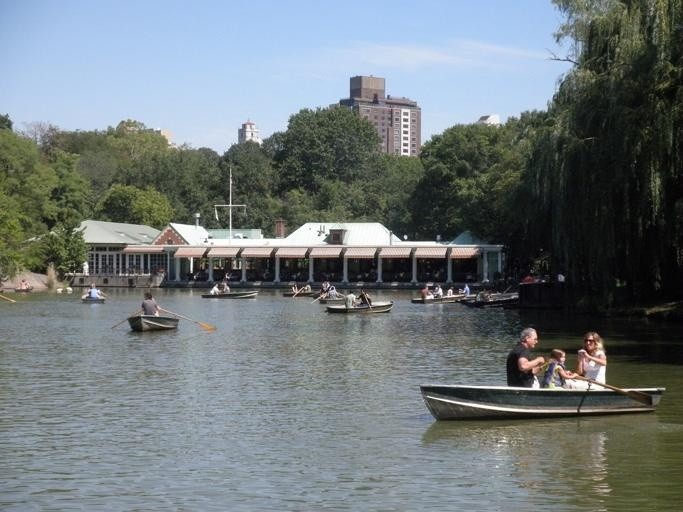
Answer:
[[542, 348, 579, 389], [217, 280, 231, 293], [507, 327, 545, 389], [291, 280, 371, 309], [421, 273, 566, 302], [209, 282, 220, 296], [573, 331, 607, 389], [19, 279, 29, 289], [142, 292, 161, 317], [88, 282, 101, 299]]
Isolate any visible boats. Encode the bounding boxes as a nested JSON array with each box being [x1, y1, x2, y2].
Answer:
[[81, 291, 106, 304], [280, 288, 395, 314], [419, 381, 665, 421], [201, 289, 260, 299], [124, 314, 180, 330], [14, 285, 34, 292], [410, 292, 519, 311]]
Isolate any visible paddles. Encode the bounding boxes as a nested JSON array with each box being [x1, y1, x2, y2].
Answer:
[[575, 375, 652, 408], [161, 309, 217, 331]]
